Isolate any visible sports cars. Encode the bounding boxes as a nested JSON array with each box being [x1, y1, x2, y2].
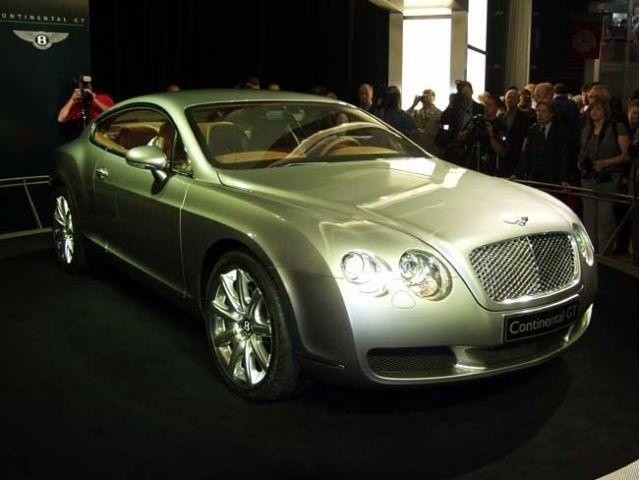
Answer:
[[46, 85, 601, 404]]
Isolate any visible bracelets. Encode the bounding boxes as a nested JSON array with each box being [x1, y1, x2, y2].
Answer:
[[489, 135, 495, 137]]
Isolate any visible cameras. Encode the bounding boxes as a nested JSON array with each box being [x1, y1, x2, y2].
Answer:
[[418, 95, 425, 101], [79, 75, 93, 103], [581, 156, 593, 171], [472, 115, 485, 127]]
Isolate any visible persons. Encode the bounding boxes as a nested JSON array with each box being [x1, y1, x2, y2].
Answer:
[[244, 76, 260, 90], [376, 85, 416, 135], [358, 84, 378, 114], [434, 80, 639, 262], [58, 76, 118, 130], [408, 89, 443, 147], [166, 84, 181, 91]]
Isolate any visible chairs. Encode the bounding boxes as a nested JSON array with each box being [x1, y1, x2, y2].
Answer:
[[114, 121, 294, 156]]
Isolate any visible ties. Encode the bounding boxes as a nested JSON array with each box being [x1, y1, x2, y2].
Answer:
[[541, 127, 545, 139]]
